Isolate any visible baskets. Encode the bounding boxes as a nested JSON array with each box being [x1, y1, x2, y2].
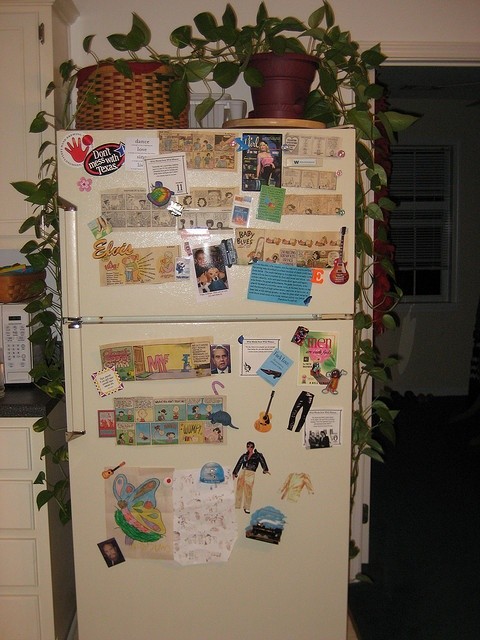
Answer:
[[76, 59, 190, 130], [0, 269, 47, 303]]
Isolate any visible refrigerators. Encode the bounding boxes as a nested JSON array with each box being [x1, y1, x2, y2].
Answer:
[[56, 129, 355, 639]]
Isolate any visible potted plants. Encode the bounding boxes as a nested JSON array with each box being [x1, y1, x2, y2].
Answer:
[[9, 0, 421, 585]]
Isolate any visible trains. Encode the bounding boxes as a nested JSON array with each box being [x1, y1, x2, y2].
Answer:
[[251, 521, 283, 541]]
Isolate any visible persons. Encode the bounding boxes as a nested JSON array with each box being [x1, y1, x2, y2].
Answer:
[[309, 432, 319, 450], [195, 250, 226, 294], [102, 543, 125, 568], [255, 142, 275, 181], [232, 441, 270, 514], [210, 345, 231, 374], [320, 431, 329, 448]]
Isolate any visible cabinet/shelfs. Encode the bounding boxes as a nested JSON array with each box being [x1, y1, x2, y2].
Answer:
[[0, 386, 76, 640]]
